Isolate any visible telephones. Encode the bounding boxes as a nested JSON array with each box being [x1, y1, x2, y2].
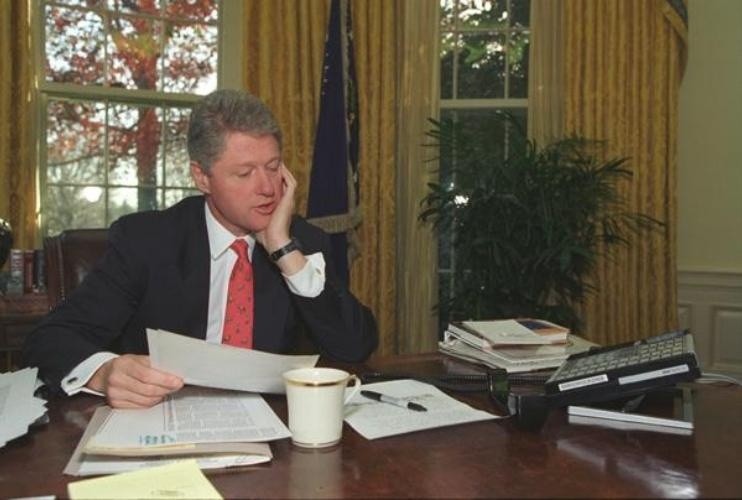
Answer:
[[485, 327, 703, 438]]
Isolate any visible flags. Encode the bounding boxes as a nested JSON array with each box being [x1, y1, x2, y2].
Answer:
[[305, 0, 365, 271]]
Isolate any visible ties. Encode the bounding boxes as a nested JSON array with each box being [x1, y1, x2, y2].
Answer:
[[222, 238, 254, 351]]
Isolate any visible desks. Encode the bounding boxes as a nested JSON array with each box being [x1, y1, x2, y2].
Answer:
[[0, 352, 741, 500], [0, 295, 65, 372]]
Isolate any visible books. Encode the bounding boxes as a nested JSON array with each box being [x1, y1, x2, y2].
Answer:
[[11, 248, 48, 290], [62, 385, 293, 477], [437, 317, 604, 374]]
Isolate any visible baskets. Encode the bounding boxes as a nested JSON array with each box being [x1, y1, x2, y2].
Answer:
[[0, 292, 51, 317]]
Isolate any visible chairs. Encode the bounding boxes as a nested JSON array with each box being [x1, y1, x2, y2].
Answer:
[[43, 228, 115, 312]]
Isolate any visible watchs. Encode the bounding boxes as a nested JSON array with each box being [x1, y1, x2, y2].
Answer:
[[271, 236, 302, 261]]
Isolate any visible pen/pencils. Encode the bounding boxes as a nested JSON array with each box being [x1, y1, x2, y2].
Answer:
[[360, 390, 427, 411]]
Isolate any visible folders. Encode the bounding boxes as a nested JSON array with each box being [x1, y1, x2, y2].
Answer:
[[60, 405, 275, 478]]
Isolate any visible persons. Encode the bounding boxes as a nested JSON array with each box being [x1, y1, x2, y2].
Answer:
[[23, 88, 379, 409]]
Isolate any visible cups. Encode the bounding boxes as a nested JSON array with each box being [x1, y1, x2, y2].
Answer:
[[283, 368, 362, 448]]
